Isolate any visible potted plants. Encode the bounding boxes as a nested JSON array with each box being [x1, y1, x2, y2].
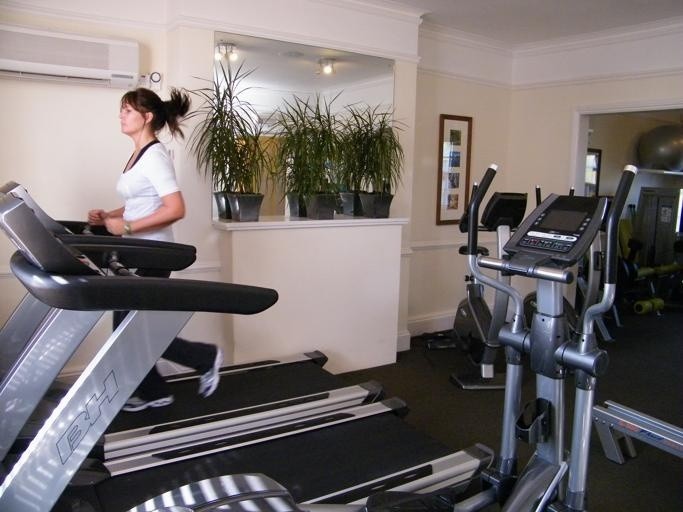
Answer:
[[177, 41, 278, 222], [267, 89, 346, 220], [333, 100, 408, 218]]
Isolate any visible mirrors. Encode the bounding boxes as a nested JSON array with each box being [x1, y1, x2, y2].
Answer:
[[211, 28, 397, 223]]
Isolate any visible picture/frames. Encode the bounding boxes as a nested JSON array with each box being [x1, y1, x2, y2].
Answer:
[[436, 113, 474, 225]]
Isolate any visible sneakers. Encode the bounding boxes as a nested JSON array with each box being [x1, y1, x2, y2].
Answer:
[[122, 392, 175, 412], [197, 346, 223, 401]]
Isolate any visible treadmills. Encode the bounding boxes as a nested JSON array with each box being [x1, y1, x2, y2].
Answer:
[[0, 192, 495, 511], [0, 183, 384, 480]]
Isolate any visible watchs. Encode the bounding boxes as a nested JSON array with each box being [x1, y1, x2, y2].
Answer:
[[124, 221, 134, 234]]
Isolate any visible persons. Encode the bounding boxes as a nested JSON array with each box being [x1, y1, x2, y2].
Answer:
[[89, 88, 222, 413]]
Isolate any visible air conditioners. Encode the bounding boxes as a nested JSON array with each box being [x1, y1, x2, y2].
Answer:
[[0, 22, 141, 91]]
